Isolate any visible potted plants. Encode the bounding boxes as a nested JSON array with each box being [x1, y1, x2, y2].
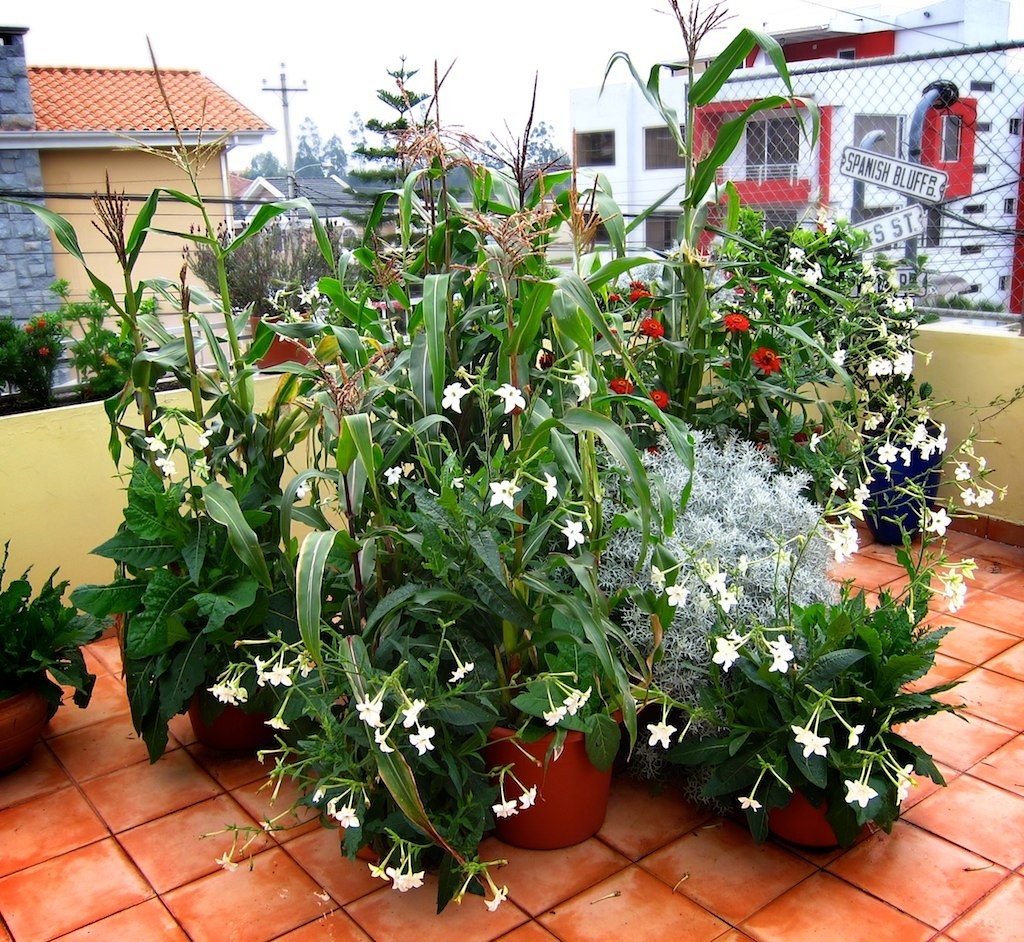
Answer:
[[68, 459, 362, 765], [830, 251, 943, 546], [703, 203, 847, 521], [0, 538, 115, 761], [48, 279, 160, 402]]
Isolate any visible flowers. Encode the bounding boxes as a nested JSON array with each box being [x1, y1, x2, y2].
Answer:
[[0, 0, 1024, 911]]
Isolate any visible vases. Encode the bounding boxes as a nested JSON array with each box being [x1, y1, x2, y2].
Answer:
[[766, 794, 864, 847], [250, 314, 312, 372], [483, 710, 623, 850]]
